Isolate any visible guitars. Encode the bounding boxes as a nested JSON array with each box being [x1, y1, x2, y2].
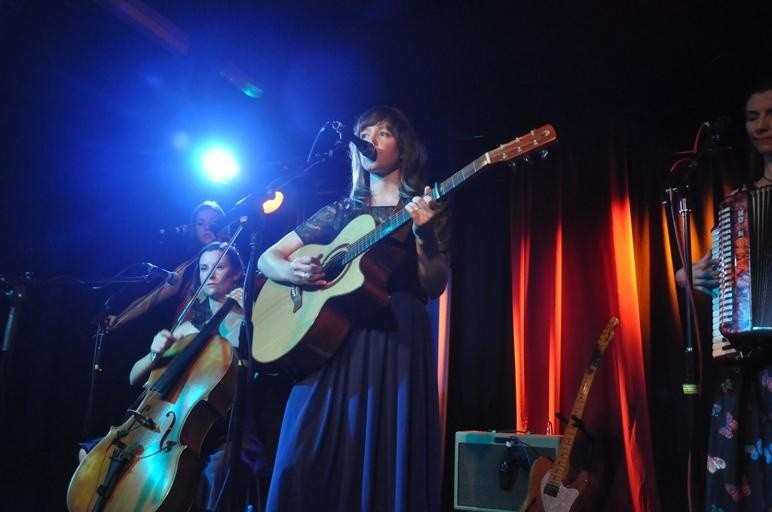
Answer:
[[519, 316, 619, 512], [251, 124, 559, 382]]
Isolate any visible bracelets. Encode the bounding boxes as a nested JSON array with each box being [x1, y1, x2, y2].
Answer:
[[150, 352, 160, 361]]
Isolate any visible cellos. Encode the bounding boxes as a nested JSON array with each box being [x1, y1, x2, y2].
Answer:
[[66, 271, 267, 512]]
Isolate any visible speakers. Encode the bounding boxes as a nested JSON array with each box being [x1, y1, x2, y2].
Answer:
[[454, 430, 603, 512]]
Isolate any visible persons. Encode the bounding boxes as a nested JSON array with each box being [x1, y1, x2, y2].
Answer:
[[101, 198, 232, 336], [76, 240, 256, 511], [673, 84, 771, 512], [256, 104, 457, 512]]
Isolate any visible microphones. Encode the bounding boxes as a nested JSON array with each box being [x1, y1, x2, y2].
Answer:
[[149, 262, 182, 287], [166, 224, 189, 241], [334, 120, 377, 161]]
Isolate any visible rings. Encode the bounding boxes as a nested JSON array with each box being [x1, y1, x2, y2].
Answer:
[[302, 271, 307, 278], [414, 206, 421, 211]]
